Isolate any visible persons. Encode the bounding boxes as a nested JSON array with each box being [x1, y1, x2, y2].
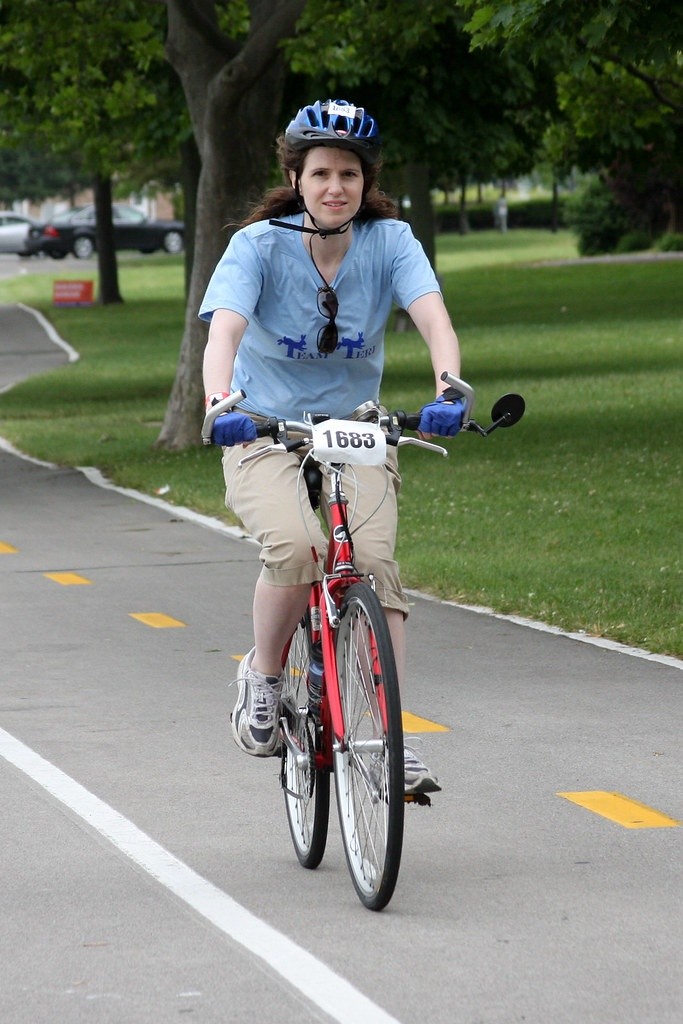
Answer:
[[192, 99, 469, 795]]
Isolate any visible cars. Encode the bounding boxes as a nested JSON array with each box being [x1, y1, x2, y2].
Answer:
[[0, 211, 40, 256], [28, 202, 187, 256]]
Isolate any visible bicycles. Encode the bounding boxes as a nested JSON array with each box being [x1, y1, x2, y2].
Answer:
[[184, 367, 524, 915]]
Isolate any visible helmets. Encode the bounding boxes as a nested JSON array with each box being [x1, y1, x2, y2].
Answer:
[[284, 98, 383, 164]]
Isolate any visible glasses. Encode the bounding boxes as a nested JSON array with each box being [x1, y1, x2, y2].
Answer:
[[316, 286, 339, 353]]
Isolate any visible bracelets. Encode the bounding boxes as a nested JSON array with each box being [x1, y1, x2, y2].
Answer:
[[204, 393, 230, 407]]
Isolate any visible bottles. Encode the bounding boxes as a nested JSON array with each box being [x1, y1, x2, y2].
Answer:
[[306, 639, 325, 714]]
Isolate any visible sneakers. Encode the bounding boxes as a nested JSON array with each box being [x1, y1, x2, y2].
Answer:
[[370, 738, 440, 798], [231, 646, 285, 756]]
